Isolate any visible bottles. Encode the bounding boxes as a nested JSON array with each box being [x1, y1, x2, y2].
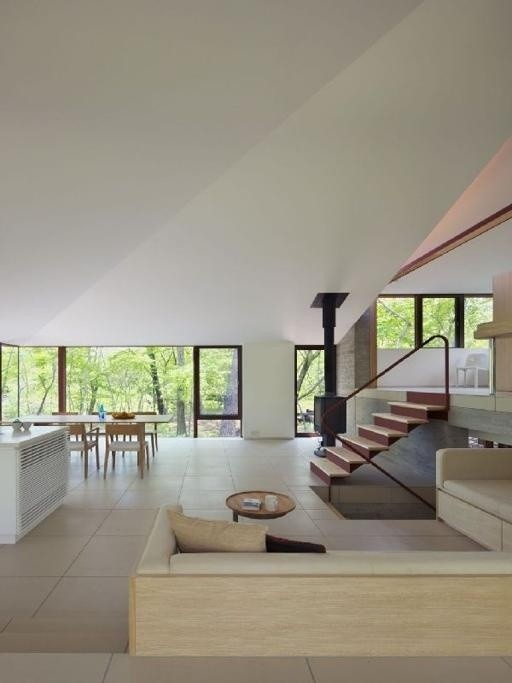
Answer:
[[98, 405, 106, 421]]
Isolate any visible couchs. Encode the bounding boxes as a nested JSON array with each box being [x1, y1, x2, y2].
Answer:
[[128, 502, 512, 657], [433, 447, 512, 552]]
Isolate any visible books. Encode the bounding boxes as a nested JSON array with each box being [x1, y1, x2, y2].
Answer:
[[241, 498, 261, 510]]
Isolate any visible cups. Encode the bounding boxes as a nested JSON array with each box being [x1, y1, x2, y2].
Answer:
[[23, 422, 32, 432], [265, 495, 279, 512], [13, 421, 22, 432]]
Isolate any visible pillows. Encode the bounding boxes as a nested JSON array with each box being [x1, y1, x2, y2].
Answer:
[[165, 508, 270, 553], [264, 533, 327, 553]]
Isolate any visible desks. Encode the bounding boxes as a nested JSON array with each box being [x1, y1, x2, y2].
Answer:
[[0, 415, 175, 465]]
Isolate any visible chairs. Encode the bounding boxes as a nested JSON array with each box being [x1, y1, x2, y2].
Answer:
[[48, 423, 99, 480], [52, 411, 79, 442], [90, 412, 119, 456], [455, 352, 489, 388], [103, 423, 149, 480], [122, 412, 159, 458]]
